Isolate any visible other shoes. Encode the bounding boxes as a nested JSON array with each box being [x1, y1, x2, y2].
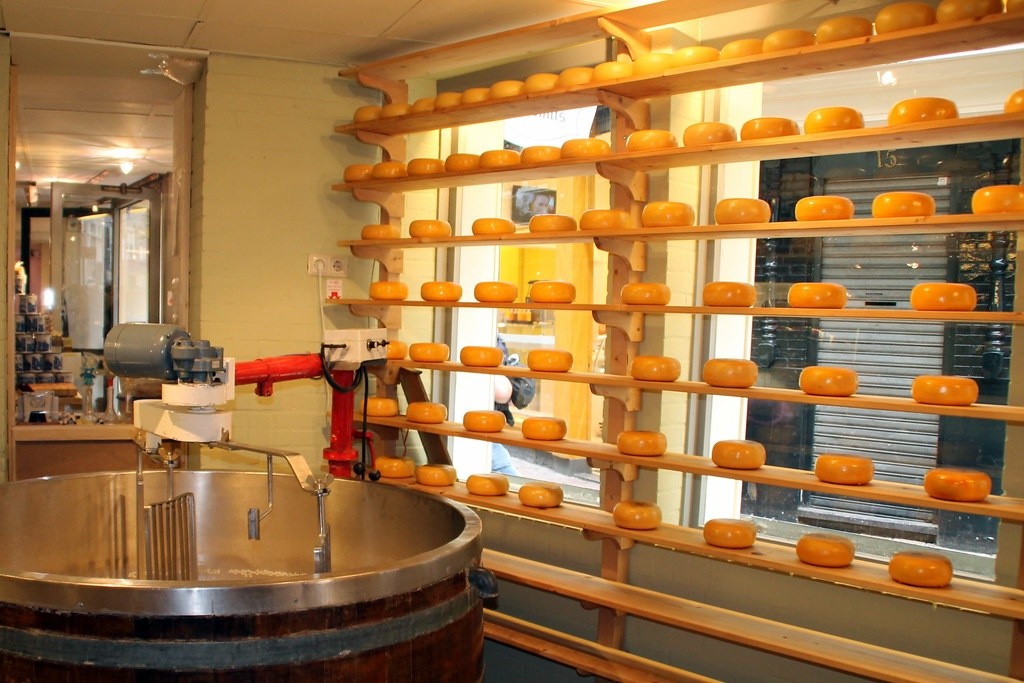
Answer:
[[493, 466, 517, 479]]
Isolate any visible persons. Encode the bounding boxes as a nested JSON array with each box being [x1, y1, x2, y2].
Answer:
[[492, 334, 518, 475], [531, 192, 550, 217]]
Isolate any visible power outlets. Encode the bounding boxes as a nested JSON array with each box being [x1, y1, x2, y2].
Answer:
[[308, 255, 349, 278]]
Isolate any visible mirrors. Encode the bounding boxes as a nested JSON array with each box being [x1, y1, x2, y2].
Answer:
[[8, 70, 193, 406]]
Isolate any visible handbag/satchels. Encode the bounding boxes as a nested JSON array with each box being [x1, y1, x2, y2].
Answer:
[[496, 340, 535, 409]]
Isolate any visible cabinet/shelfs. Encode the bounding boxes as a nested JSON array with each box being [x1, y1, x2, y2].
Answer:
[[330, 1, 1024, 683]]
